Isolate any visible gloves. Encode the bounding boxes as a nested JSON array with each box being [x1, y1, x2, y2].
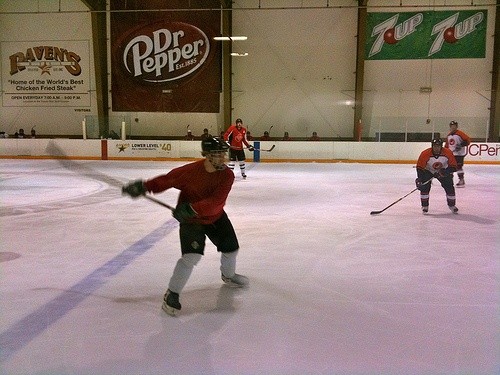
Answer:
[[249, 145, 254, 151], [172, 202, 198, 223], [437, 170, 446, 178], [225, 141, 231, 148], [416, 178, 424, 190], [128, 182, 143, 197]]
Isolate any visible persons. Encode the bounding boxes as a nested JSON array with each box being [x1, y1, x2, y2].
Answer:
[[223, 118, 256, 177], [415, 136, 460, 212], [247, 131, 254, 141], [444, 120, 471, 186], [200, 128, 222, 157], [121, 135, 249, 310], [280, 131, 291, 141], [259, 131, 272, 141], [220, 131, 225, 140], [19, 128, 26, 138], [108, 130, 120, 140], [184, 124, 195, 141], [310, 131, 320, 141]]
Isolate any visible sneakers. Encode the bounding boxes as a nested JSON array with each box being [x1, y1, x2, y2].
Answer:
[[449, 205, 458, 213], [456, 180, 466, 188], [222, 272, 248, 287], [162, 289, 181, 316], [423, 205, 428, 214], [242, 173, 246, 178]]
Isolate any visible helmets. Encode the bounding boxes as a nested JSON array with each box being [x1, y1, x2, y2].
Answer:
[[433, 138, 442, 144], [236, 119, 241, 122], [450, 121, 457, 125], [188, 130, 191, 132], [201, 136, 229, 171]]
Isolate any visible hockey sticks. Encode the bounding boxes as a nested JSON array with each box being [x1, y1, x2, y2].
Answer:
[[413, 144, 464, 168], [370, 177, 435, 215], [187, 124, 190, 132], [269, 125, 274, 134], [231, 145, 275, 152], [48, 140, 176, 211]]
